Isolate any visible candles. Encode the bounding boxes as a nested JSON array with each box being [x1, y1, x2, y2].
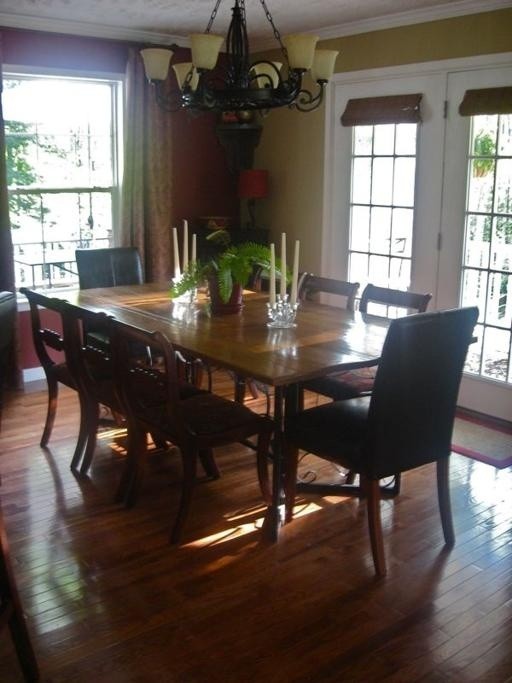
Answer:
[[171, 221, 198, 276], [268, 232, 301, 308]]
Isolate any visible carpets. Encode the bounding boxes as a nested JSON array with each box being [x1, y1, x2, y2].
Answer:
[[446, 410, 512, 470]]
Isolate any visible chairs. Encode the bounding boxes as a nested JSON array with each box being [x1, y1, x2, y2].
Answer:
[[285, 307, 480, 580], [17, 246, 434, 547]]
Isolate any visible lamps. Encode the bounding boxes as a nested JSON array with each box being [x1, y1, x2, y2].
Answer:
[[237, 168, 267, 231], [133, 0, 340, 127]]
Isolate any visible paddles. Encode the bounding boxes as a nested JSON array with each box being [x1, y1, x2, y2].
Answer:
[[446, 410, 512, 470]]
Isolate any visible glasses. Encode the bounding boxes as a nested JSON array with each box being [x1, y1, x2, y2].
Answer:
[[133, 0, 340, 127], [237, 168, 267, 231]]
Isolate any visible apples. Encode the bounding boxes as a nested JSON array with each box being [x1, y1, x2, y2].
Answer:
[[171, 221, 198, 276], [268, 232, 301, 308]]
